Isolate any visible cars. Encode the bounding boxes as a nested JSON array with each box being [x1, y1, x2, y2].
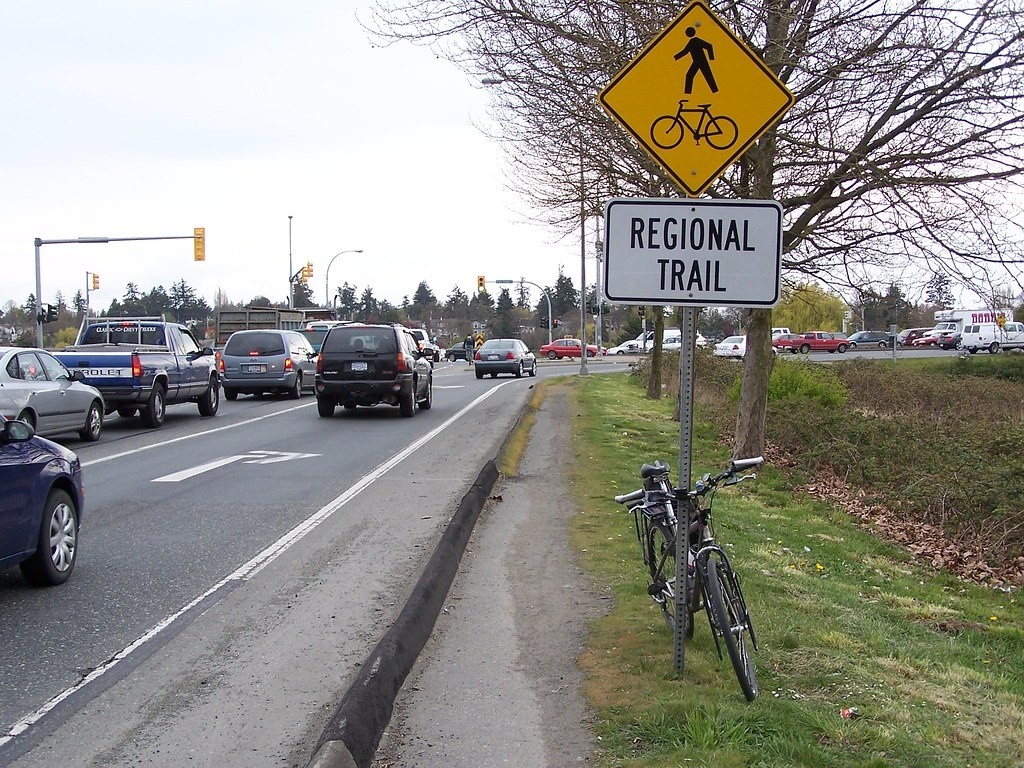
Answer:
[[407, 327, 447, 363], [541, 328, 961, 360], [0, 346, 107, 443], [445, 341, 477, 363], [0, 413, 84, 587], [474, 339, 538, 380]]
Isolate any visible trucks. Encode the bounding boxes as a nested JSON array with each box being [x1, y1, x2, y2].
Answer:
[[932, 309, 1015, 332]]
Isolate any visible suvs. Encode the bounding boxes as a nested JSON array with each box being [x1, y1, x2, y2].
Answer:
[[314, 322, 434, 418]]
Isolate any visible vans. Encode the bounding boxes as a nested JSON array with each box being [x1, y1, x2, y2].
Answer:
[[220, 328, 320, 400], [291, 319, 380, 354], [962, 323, 1024, 354]]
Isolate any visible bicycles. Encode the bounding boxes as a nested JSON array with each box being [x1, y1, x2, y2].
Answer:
[[612, 456, 772, 701], [464, 346, 475, 365]]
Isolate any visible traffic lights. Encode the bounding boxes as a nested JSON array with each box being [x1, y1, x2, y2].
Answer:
[[302, 269, 307, 284], [42, 307, 47, 324], [477, 275, 485, 293], [47, 304, 58, 323], [93, 275, 100, 289], [308, 262, 313, 278], [540, 317, 558, 328]]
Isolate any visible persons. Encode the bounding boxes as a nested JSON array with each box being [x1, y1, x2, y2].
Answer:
[[464, 334, 475, 364]]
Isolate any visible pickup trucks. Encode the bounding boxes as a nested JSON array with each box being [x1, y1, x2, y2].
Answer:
[[30, 313, 221, 429], [776, 331, 850, 354]]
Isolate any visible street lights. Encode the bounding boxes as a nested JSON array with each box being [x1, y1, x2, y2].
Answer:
[[480, 76, 589, 378], [325, 250, 363, 308]]
